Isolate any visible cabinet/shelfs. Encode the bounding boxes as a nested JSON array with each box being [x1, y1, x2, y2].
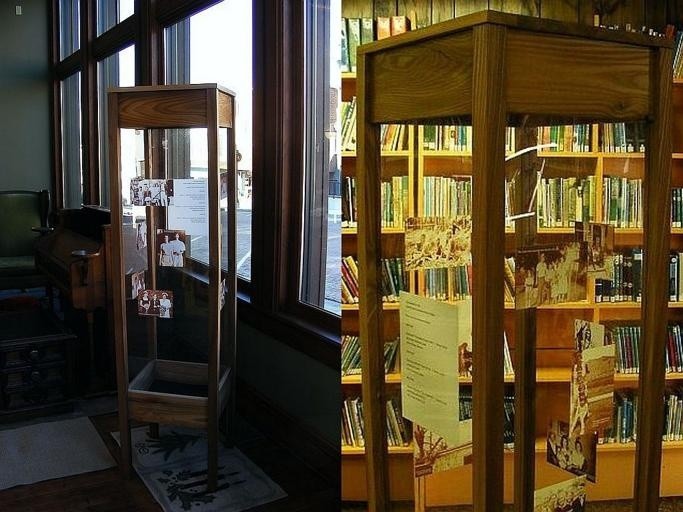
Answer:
[[102, 84, 239, 493], [0, 306, 78, 413]]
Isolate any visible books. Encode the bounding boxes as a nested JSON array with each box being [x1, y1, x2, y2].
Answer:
[[340, 172, 516, 229], [391, 15, 411, 38], [595, 253, 681, 303], [406, 252, 517, 305], [592, 14, 682, 86], [504, 115, 627, 157], [341, 15, 350, 73], [341, 396, 412, 448], [341, 256, 405, 305], [341, 98, 474, 157], [535, 171, 681, 229], [607, 324, 682, 373], [359, 17, 376, 46], [597, 390, 682, 444], [341, 336, 400, 377], [376, 15, 391, 41], [347, 18, 361, 73]]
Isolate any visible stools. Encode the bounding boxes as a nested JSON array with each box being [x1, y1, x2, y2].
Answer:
[[0, 294, 42, 339]]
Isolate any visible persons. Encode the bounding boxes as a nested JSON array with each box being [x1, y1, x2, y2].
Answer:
[[555, 491, 571, 511], [573, 483, 585, 511], [537, 503, 548, 512], [571, 354, 588, 434], [158, 234, 172, 266], [130, 181, 173, 206], [149, 293, 159, 313], [555, 433, 569, 469], [548, 432, 558, 465], [159, 292, 171, 317], [577, 322, 592, 351], [136, 280, 141, 295], [567, 441, 584, 473], [565, 485, 581, 512], [168, 233, 184, 267], [524, 253, 565, 307], [548, 495, 556, 511], [139, 291, 148, 314]]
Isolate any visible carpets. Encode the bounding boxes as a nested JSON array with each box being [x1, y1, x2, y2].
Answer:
[[107, 417, 291, 511], [0, 413, 117, 494]]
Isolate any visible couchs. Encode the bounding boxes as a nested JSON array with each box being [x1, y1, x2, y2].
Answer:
[[0, 190, 53, 303]]
